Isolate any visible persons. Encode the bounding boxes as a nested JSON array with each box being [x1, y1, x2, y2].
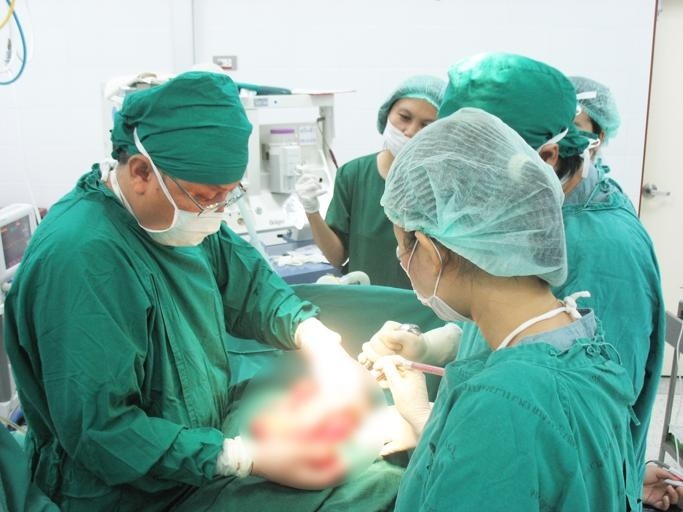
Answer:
[[3, 69, 356, 512]]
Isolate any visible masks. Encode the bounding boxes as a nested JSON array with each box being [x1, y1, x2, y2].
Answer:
[[123, 185, 227, 246], [383, 117, 410, 156], [401, 240, 469, 321]]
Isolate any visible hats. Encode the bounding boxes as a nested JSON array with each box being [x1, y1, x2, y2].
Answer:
[[439, 55, 599, 157], [111, 71, 252, 184]]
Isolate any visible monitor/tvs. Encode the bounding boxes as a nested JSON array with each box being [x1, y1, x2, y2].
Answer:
[[0, 203, 38, 283]]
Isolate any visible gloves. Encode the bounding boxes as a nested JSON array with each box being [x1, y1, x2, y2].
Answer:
[[296, 175, 326, 214], [297, 317, 347, 362], [358, 320, 463, 433]]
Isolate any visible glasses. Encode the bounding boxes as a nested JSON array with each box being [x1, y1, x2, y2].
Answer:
[[171, 179, 247, 218]]
